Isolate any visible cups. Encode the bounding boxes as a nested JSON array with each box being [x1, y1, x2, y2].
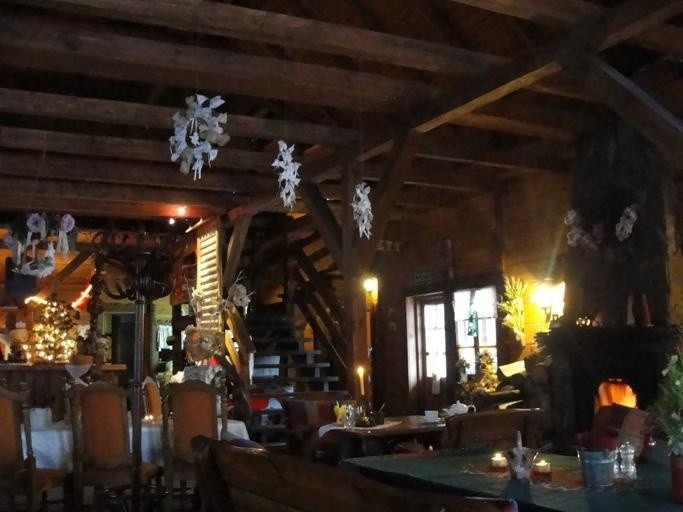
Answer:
[[424, 409, 439, 422], [335, 404, 354, 430]]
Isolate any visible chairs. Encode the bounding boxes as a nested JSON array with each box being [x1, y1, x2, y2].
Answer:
[[1, 376, 228, 510], [272, 389, 543, 463], [579, 409, 654, 461]]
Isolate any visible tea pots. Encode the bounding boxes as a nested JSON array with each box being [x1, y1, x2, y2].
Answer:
[[441, 398, 478, 417], [612, 440, 638, 480]]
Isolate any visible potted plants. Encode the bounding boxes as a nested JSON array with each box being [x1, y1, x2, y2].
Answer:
[[648, 349, 682, 503]]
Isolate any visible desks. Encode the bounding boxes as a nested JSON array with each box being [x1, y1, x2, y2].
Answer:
[[337, 444, 682, 511], [19, 416, 250, 509]]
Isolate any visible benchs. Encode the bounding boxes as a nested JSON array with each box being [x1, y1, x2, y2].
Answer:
[[190, 431, 521, 511]]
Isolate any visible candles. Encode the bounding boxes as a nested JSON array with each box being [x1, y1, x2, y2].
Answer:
[[490, 452, 508, 466], [532, 459, 550, 472], [356, 366, 366, 395]]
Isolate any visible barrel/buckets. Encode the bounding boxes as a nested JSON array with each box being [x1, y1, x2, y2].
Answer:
[[578, 448, 615, 488]]
